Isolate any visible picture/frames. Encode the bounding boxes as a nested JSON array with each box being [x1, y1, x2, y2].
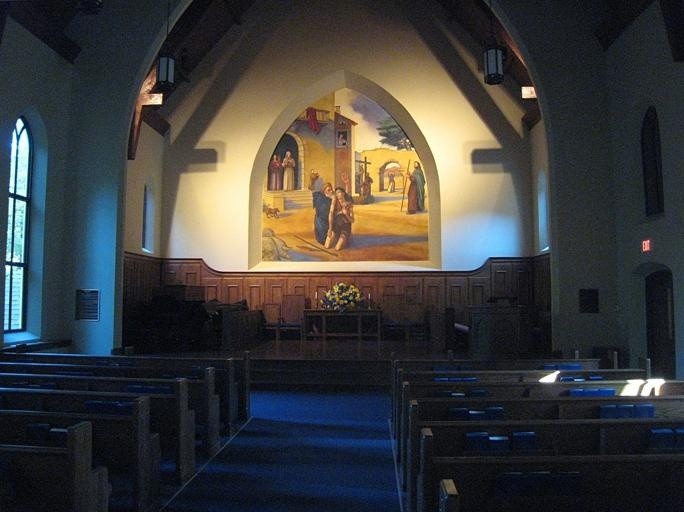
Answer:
[[248, 68, 441, 271]]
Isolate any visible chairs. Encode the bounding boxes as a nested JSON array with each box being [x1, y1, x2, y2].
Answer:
[[275, 294, 306, 341], [378, 294, 406, 342]]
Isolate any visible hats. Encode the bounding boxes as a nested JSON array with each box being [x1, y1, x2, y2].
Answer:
[[320, 183, 332, 192]]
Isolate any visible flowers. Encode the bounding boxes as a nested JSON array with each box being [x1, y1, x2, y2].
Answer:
[[318, 281, 367, 314]]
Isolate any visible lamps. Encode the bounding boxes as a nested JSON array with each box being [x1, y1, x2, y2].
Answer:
[[155, 0, 174, 89], [482, 0, 504, 84]]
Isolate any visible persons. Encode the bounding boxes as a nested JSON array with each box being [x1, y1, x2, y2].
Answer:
[[387, 172, 396, 194], [406, 160, 426, 214], [307, 164, 374, 252], [269, 150, 296, 192]]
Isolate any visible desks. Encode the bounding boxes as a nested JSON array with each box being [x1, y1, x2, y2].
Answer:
[[302, 308, 382, 340]]
